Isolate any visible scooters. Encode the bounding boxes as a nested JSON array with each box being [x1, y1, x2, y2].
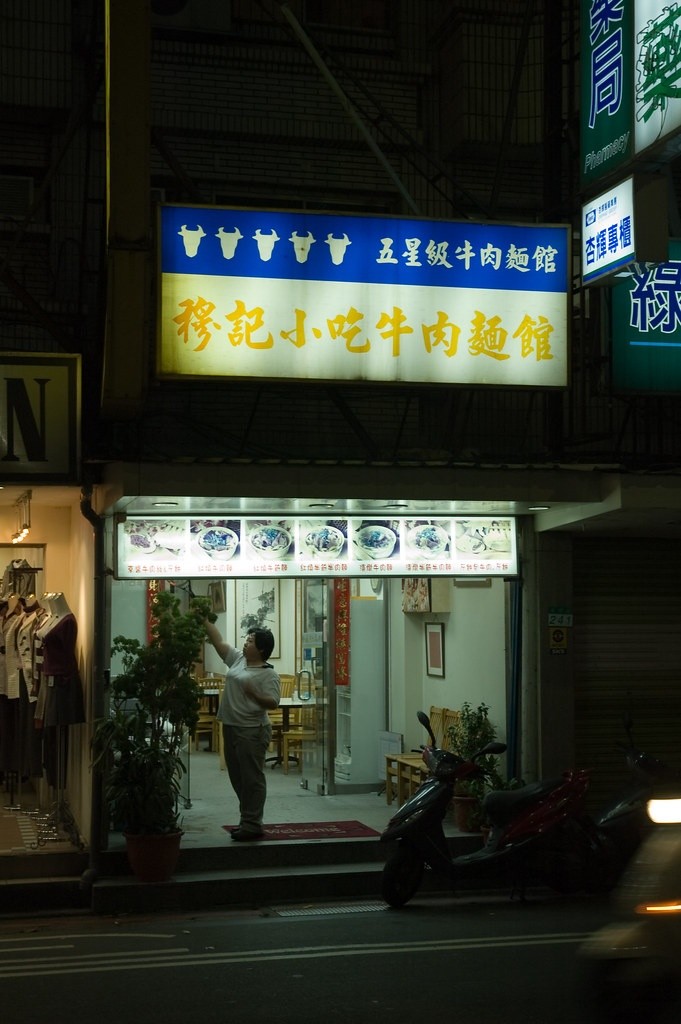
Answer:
[[372, 710, 601, 908], [587, 713, 681, 899]]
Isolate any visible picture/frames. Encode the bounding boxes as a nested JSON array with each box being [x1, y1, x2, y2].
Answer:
[[424, 622, 445, 679]]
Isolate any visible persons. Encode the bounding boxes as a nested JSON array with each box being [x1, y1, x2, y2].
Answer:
[[0, 559, 86, 728], [194, 608, 281, 840]]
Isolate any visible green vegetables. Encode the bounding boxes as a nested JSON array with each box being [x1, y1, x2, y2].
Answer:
[[203, 528, 438, 551]]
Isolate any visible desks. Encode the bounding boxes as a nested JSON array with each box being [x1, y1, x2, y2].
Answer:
[[219, 698, 327, 770], [199, 687, 223, 752]]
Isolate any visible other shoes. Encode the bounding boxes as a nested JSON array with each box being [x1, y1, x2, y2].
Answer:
[[231, 830, 263, 840]]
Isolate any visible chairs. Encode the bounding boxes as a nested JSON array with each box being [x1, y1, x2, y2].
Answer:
[[385, 706, 470, 810], [188, 673, 323, 775]]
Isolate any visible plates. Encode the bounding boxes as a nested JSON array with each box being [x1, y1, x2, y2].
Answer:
[[457, 539, 486, 554], [483, 533, 509, 552], [126, 534, 156, 553]]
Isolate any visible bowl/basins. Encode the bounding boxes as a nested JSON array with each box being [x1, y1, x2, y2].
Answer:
[[197, 527, 238, 561], [303, 526, 344, 559], [249, 526, 291, 557], [407, 525, 447, 559], [356, 526, 396, 559]]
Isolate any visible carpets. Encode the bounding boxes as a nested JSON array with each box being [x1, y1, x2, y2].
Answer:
[[222, 821, 382, 841]]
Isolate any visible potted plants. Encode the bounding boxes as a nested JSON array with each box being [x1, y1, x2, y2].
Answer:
[[447, 701, 499, 832], [87, 705, 183, 882]]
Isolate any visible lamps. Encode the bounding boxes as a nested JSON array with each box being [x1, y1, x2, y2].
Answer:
[[11, 489, 33, 544]]
